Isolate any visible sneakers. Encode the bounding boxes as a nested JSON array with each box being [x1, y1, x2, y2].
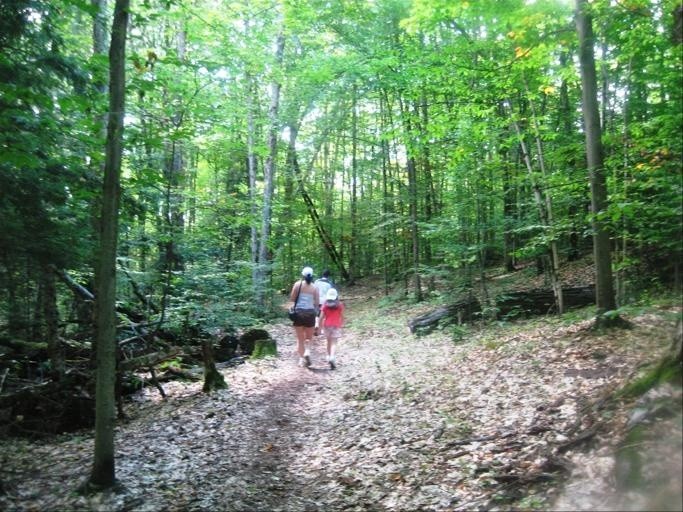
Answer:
[[313, 325, 319, 336], [297, 361, 304, 367], [328, 359, 336, 369], [303, 353, 312, 367]]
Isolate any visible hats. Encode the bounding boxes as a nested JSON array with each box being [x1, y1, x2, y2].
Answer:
[[326, 288, 337, 300], [301, 267, 313, 278]]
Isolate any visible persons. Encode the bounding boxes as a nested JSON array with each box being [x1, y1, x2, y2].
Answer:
[[316, 287, 345, 369], [290, 266, 320, 368], [313, 268, 338, 337]]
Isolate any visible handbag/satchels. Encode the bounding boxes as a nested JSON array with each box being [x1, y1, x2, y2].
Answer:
[[287, 307, 297, 322]]
